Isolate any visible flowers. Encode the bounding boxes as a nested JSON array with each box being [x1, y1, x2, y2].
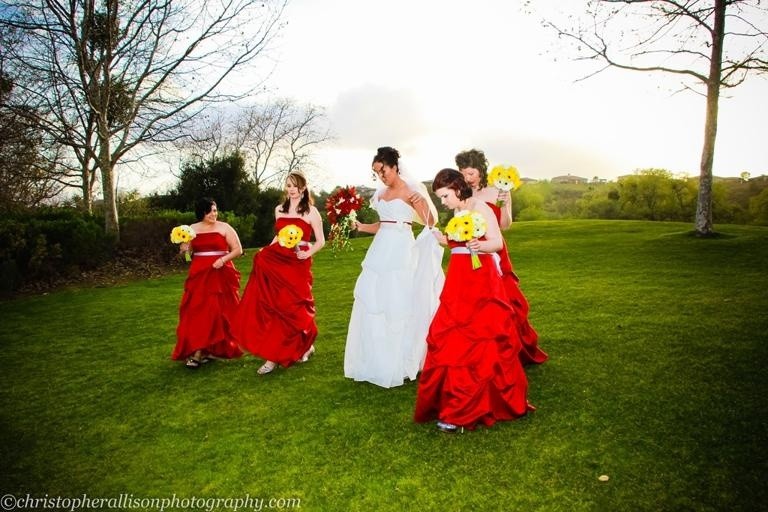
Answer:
[[277, 224, 304, 251], [486, 166, 521, 208], [170, 224, 196, 263], [324, 182, 365, 259], [444, 210, 487, 271]]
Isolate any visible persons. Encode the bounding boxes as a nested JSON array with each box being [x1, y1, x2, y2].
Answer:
[[414, 169, 535, 432], [455, 149, 548, 366], [344, 147, 446, 389], [171, 197, 243, 369], [228, 172, 326, 375]]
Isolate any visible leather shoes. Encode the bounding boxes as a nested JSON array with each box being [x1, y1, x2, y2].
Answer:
[[296, 344, 315, 363], [256, 361, 279, 376]]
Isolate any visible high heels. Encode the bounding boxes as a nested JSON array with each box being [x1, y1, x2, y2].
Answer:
[[436, 422, 465, 434]]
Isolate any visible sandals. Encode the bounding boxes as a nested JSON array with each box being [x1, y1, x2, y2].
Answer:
[[184, 359, 201, 370], [200, 356, 217, 365]]
